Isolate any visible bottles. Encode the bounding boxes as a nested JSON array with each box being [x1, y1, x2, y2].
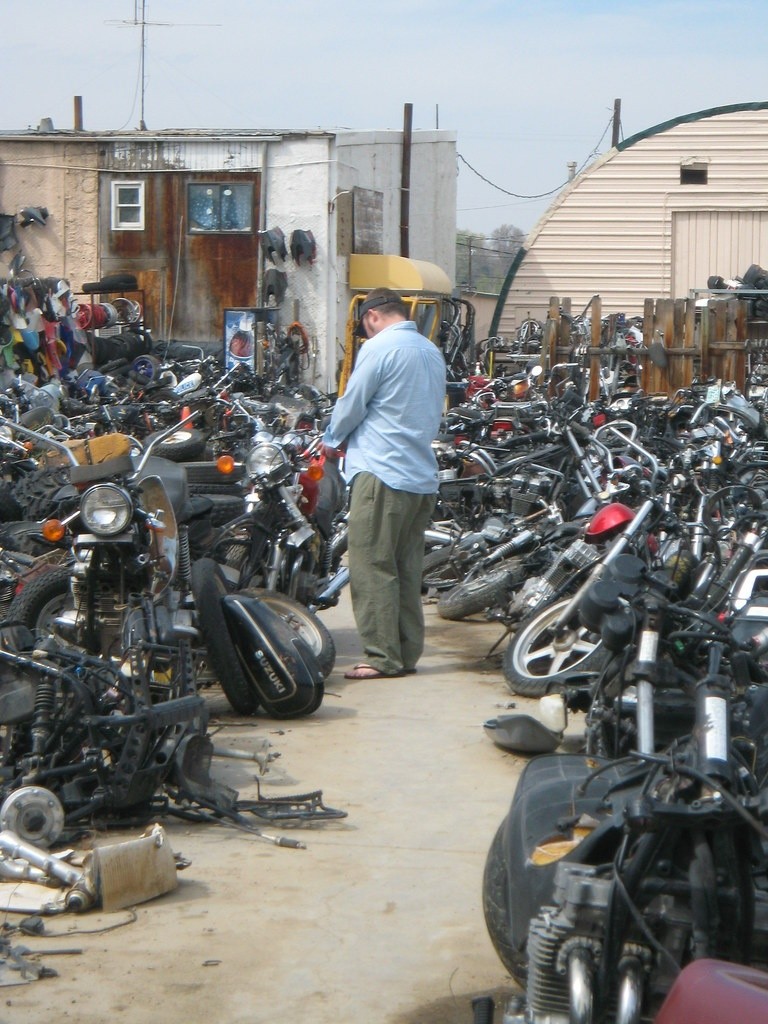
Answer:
[[228, 318, 254, 379]]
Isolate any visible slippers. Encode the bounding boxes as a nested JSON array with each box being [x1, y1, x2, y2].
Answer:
[[344, 664, 416, 679]]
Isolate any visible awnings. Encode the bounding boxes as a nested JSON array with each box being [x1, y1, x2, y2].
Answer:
[[350, 254, 458, 295]]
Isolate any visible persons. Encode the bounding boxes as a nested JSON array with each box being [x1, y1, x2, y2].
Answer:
[[315, 287, 445, 679]]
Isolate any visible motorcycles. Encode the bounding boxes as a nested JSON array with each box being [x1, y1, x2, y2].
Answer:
[[1, 332, 767, 1024]]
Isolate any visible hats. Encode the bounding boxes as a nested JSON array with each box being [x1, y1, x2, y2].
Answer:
[[353, 297, 402, 339]]
